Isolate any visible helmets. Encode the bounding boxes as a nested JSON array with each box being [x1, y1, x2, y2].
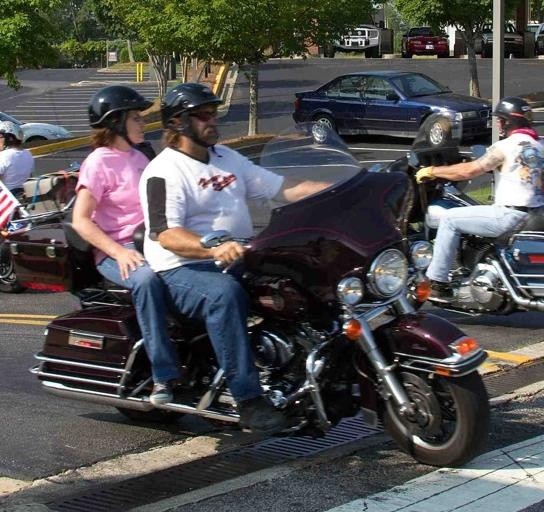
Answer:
[[160, 80, 223, 129], [85, 82, 154, 128], [490, 95, 535, 128], [0, 116, 23, 142]]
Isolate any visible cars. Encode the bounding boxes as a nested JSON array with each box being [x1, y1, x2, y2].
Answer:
[[291, 67, 491, 148], [0, 110, 73, 152], [323, 6, 543, 59]]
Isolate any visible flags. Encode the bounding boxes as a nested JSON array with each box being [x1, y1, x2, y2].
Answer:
[[0, 181, 21, 229]]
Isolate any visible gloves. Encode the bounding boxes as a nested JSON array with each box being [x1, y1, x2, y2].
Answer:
[[414, 164, 437, 186]]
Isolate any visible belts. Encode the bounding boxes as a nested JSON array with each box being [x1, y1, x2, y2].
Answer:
[[499, 199, 543, 214]]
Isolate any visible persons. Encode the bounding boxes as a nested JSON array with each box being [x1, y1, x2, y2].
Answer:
[[71, 85, 183, 405], [0, 121, 34, 198], [415, 96, 544, 297], [138, 84, 337, 437]]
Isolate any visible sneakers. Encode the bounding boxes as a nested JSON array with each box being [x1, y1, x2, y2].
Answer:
[[424, 278, 449, 298], [237, 395, 288, 435], [144, 370, 176, 409]]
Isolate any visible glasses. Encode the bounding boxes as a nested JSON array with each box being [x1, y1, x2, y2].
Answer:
[[172, 109, 221, 121]]
